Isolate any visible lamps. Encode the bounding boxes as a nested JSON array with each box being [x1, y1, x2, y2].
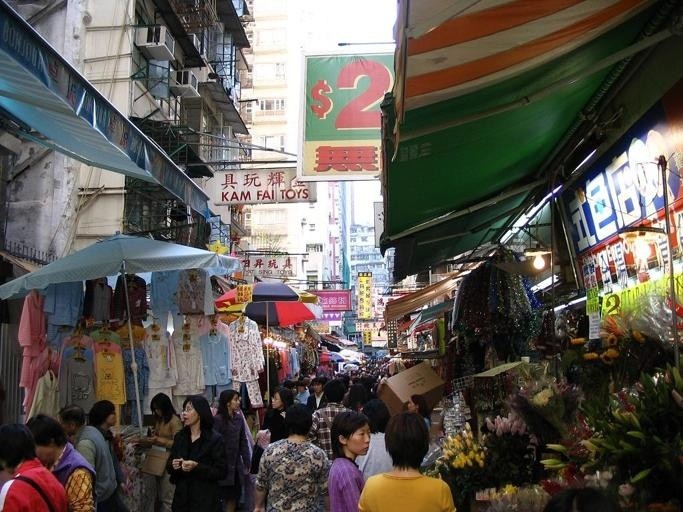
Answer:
[[617, 224, 666, 259], [524, 246, 554, 272]]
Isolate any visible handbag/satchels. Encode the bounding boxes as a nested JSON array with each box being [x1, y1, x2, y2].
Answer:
[[140, 419, 172, 477], [249, 440, 264, 474]]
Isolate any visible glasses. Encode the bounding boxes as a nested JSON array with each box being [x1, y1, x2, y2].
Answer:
[[181, 407, 193, 411], [270, 397, 280, 401]]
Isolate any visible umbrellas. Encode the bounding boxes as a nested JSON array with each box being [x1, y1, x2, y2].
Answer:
[[214, 281, 319, 399], [0, 231, 244, 431], [242, 303, 324, 326]]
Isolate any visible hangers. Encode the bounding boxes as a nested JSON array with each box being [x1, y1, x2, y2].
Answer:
[[34, 315, 273, 389]]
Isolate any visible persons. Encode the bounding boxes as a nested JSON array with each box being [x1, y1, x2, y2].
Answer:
[[310, 380, 353, 461], [407, 395, 431, 431], [26, 413, 97, 512], [252, 390, 293, 467], [253, 403, 332, 511], [307, 378, 328, 411], [296, 380, 310, 402], [58, 404, 118, 503], [0, 424, 67, 511], [166, 395, 227, 511], [88, 401, 133, 511], [355, 399, 395, 482], [326, 411, 371, 512], [212, 390, 251, 511], [349, 383, 367, 411], [138, 393, 182, 511], [357, 412, 458, 511]]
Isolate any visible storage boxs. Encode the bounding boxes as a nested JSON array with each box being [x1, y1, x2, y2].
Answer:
[[380, 363, 447, 422]]
